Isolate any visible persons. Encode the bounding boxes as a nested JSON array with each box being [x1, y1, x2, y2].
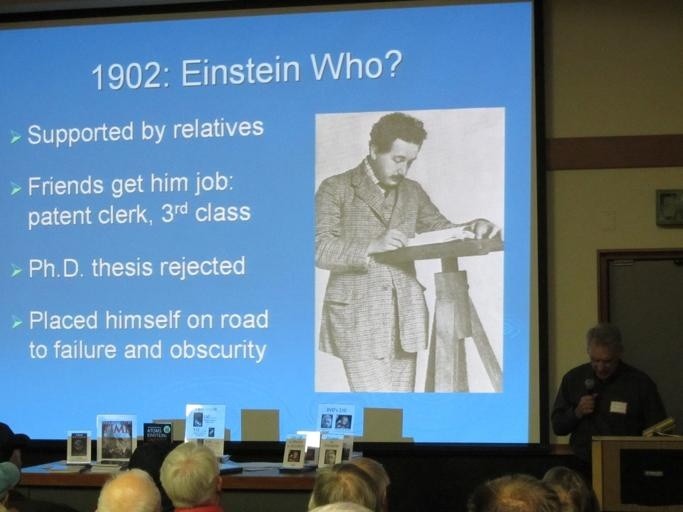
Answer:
[[466, 473, 561, 512], [94, 468, 163, 512], [429, 461, 481, 498], [542, 467, 600, 512], [0, 422, 80, 512], [315, 112, 504, 393], [160, 442, 223, 512], [349, 457, 391, 512], [552, 322, 672, 502], [311, 502, 374, 512], [308, 463, 381, 512], [128, 442, 182, 512], [395, 472, 471, 512], [0, 461, 20, 512]]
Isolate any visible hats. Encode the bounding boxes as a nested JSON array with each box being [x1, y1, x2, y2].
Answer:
[[0, 461, 20, 493], [0, 422, 29, 451]]
